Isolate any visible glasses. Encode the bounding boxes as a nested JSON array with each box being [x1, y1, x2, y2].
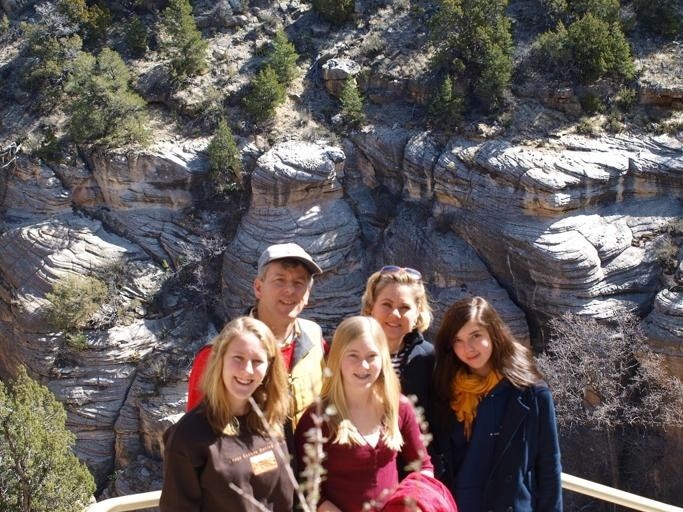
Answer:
[[374, 265, 423, 288]]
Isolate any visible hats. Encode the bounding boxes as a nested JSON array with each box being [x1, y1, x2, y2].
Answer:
[[257, 243, 323, 276]]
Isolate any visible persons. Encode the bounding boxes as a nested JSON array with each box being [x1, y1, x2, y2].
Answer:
[[292, 316, 436, 512], [356, 265, 436, 445], [186, 241, 330, 437], [425, 296, 564, 512], [157, 318, 301, 511]]
